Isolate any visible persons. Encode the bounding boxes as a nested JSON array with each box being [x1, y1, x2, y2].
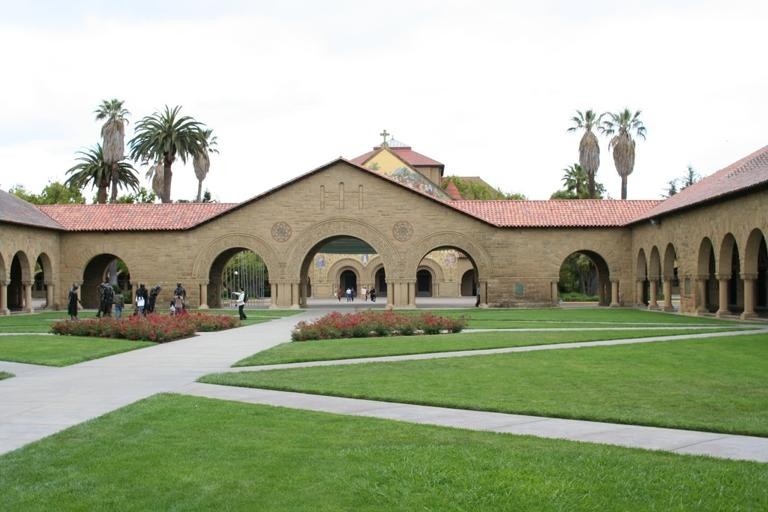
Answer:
[[231, 288, 247, 320], [113, 290, 124, 319], [148, 285, 161, 312], [361, 286, 377, 302], [135, 290, 145, 314], [67, 282, 81, 320], [173, 282, 186, 299], [175, 294, 184, 317], [96, 277, 114, 318], [336, 286, 344, 302], [136, 283, 149, 313], [346, 286, 354, 302], [169, 300, 175, 315]]
